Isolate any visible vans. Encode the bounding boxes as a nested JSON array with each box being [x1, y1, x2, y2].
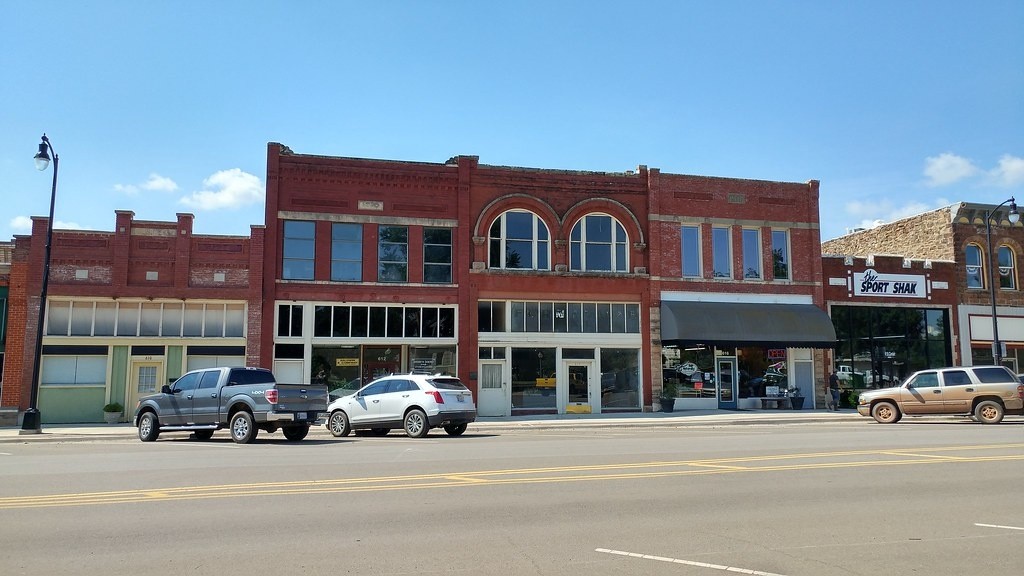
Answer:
[[601, 366, 639, 398]]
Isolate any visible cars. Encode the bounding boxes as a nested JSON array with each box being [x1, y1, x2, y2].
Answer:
[[836, 364, 899, 388], [328, 375, 379, 402], [662, 366, 779, 399]]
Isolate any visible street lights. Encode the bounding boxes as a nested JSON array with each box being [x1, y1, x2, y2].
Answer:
[[17, 134, 60, 437], [538, 351, 543, 378], [985, 195, 1020, 365]]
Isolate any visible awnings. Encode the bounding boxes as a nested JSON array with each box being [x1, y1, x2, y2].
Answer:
[[660, 300, 838, 350]]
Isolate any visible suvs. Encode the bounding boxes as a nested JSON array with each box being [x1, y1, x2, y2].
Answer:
[[856, 362, 1024, 425], [324, 371, 478, 439]]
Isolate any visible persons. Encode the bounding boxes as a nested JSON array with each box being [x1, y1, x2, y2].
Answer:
[[830, 369, 844, 411]]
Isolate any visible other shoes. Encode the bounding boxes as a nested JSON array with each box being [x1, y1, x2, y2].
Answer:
[[835, 407, 840, 411], [827, 402, 832, 409]]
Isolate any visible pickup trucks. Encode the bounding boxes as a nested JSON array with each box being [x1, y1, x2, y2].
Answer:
[[133, 366, 331, 445], [536, 372, 587, 395]]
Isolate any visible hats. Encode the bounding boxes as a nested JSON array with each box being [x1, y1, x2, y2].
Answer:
[[833, 368, 838, 373]]
